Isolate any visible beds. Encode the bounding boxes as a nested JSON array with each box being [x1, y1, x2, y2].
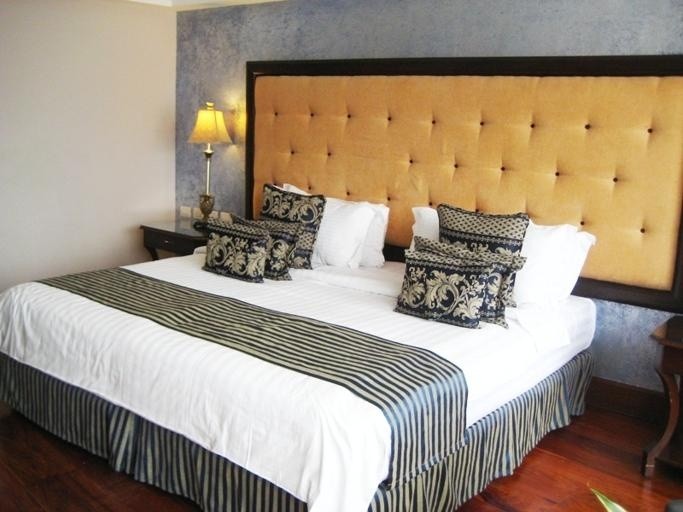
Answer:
[[0, 253, 597, 512]]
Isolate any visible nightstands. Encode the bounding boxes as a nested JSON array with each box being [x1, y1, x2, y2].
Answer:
[[139, 216, 209, 261], [643, 314, 683, 478]]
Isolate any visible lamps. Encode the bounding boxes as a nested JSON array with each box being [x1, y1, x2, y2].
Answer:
[[188, 102, 233, 231]]
